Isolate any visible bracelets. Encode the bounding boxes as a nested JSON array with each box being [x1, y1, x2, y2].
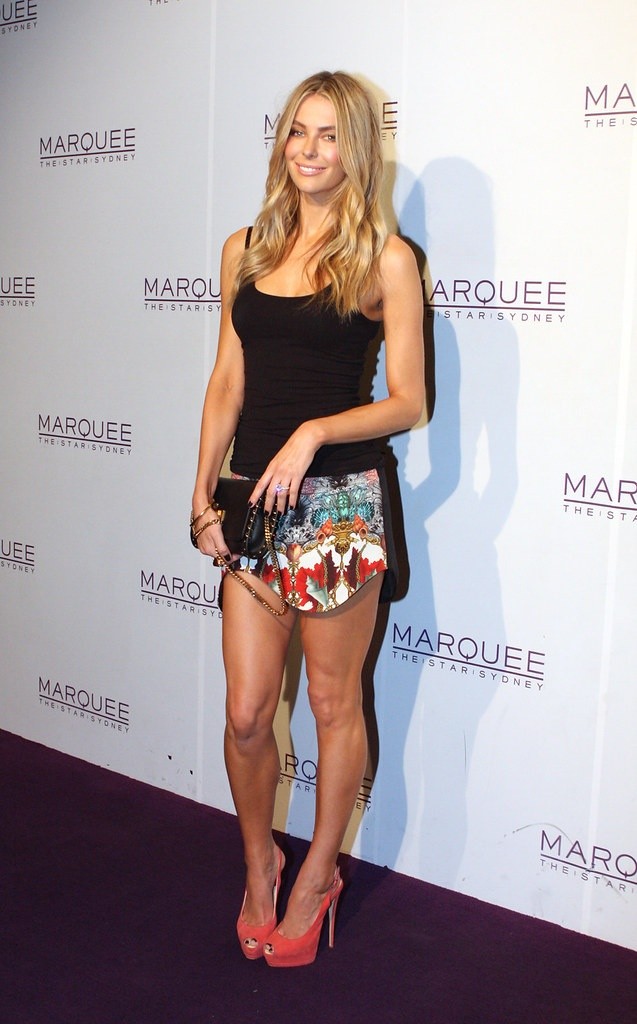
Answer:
[[193, 519, 222, 541], [190, 498, 217, 528]]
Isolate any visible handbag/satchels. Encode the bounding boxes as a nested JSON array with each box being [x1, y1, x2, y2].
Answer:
[[190, 477, 289, 617]]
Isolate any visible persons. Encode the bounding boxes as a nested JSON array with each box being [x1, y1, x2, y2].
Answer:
[[189, 73, 426, 966]]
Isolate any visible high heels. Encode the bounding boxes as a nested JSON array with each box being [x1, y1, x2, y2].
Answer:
[[237, 847, 285, 959], [264, 868, 343, 967]]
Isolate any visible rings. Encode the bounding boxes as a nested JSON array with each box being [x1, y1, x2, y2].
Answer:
[[276, 484, 289, 492]]
